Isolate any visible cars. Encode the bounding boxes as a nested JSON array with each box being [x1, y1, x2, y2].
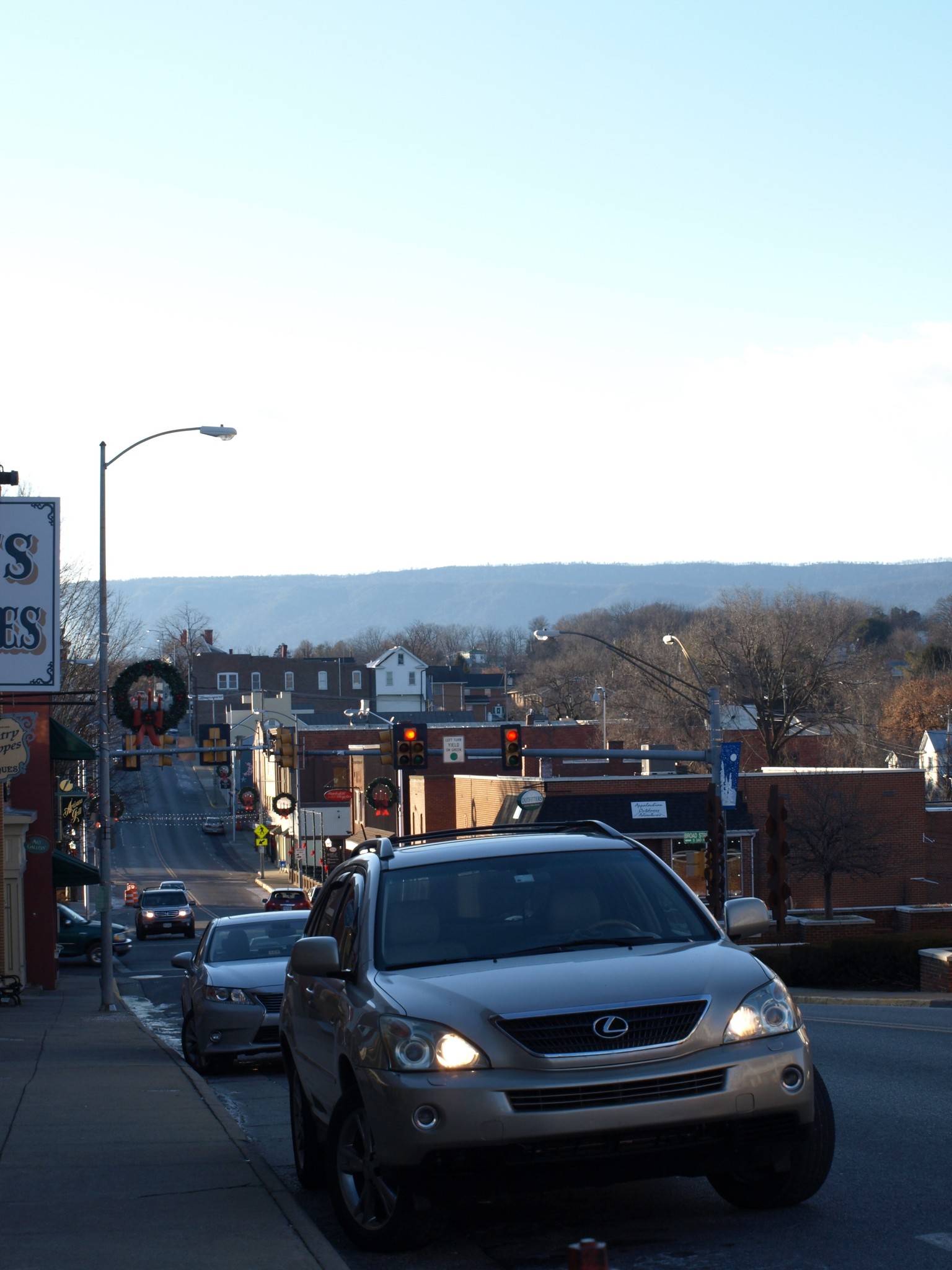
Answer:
[[201, 817, 224, 835], [165, 728, 180, 742], [171, 885, 321, 1076], [155, 881, 190, 897], [57, 902, 133, 967], [135, 692, 147, 701], [113, 750, 124, 769]]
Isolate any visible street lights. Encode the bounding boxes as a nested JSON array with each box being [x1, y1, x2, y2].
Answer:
[[222, 723, 264, 879], [533, 628, 726, 914], [140, 627, 176, 701], [344, 709, 407, 901], [99, 428, 242, 1007], [187, 694, 218, 785]]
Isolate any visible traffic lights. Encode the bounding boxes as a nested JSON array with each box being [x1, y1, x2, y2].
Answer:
[[393, 724, 429, 771], [157, 732, 174, 771], [278, 726, 298, 770], [500, 723, 523, 771]]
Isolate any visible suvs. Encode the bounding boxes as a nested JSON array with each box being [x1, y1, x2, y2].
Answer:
[[133, 886, 196, 940], [275, 819, 836, 1258]]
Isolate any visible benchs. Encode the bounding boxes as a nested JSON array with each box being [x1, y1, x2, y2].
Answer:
[[0, 973, 25, 1007]]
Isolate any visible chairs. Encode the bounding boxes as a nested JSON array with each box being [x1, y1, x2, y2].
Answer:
[[385, 897, 471, 968], [294, 894, 301, 900], [548, 888, 603, 938], [276, 893, 283, 899]]
